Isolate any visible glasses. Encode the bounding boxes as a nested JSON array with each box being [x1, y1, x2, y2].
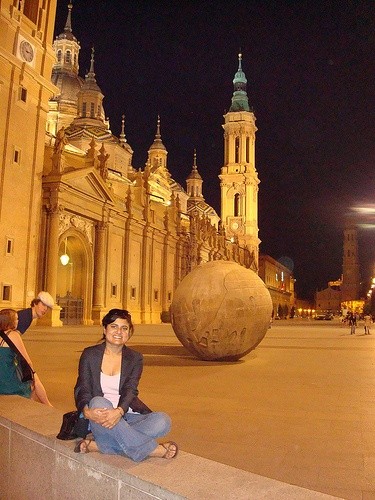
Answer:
[[109, 309, 129, 318]]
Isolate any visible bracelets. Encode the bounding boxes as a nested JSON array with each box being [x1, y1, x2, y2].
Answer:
[[116, 408, 124, 416]]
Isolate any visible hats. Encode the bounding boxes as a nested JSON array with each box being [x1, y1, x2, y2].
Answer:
[[38, 290, 54, 310]]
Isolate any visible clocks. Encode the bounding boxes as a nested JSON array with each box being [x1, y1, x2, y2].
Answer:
[[21, 40, 34, 63]]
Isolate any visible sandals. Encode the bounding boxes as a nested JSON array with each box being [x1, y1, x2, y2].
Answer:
[[73, 437, 99, 455], [144, 440, 179, 461]]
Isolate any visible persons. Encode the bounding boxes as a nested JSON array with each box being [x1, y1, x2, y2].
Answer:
[[14, 291, 54, 335], [349, 313, 357, 335], [74, 309, 178, 462], [0, 309, 53, 407], [364, 313, 371, 335], [340, 311, 375, 326], [54, 126, 69, 153]]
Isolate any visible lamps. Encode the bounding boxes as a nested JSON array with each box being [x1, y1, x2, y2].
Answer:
[[60, 237, 69, 266]]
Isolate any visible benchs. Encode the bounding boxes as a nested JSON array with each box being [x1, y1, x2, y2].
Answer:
[[0, 393, 344, 500]]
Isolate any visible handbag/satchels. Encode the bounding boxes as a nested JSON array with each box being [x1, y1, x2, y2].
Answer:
[[56, 410, 77, 442], [19, 356, 36, 382]]
